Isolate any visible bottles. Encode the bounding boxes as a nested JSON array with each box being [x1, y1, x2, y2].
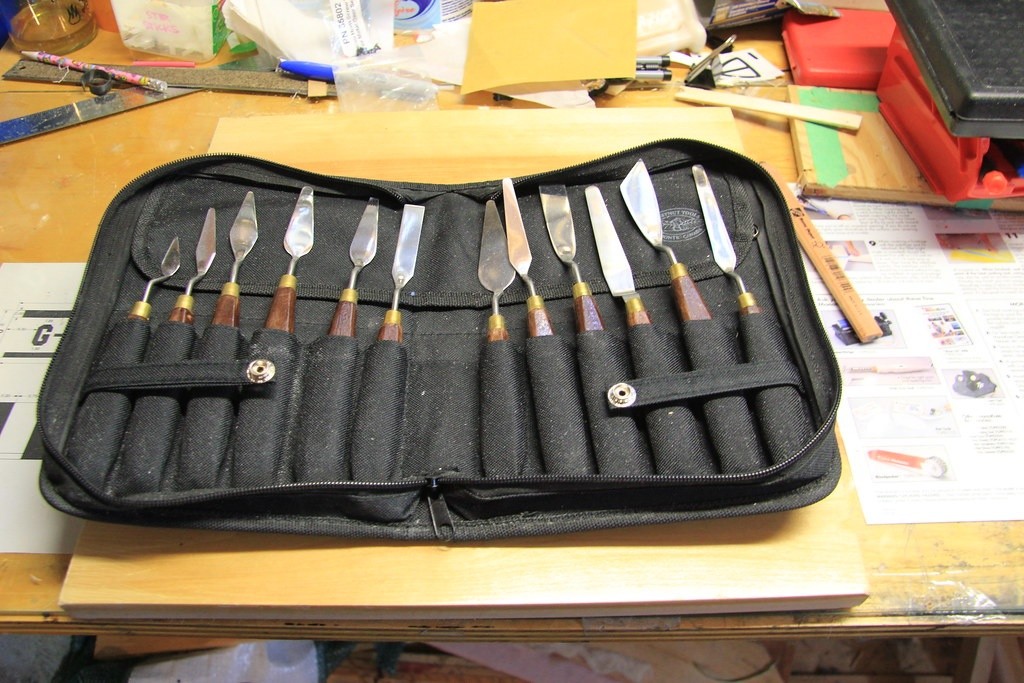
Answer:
[[977, 153, 1008, 196]]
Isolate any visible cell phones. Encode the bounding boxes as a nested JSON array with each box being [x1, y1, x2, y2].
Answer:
[[687, 35, 737, 79]]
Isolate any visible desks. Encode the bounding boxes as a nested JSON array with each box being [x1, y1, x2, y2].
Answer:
[[1, 3, 1024, 683]]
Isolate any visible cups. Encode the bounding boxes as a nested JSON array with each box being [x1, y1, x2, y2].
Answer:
[[0, 0, 97, 55]]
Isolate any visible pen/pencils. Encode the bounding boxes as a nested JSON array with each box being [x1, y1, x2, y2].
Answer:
[[20, 49, 169, 96], [132, 59, 197, 69]]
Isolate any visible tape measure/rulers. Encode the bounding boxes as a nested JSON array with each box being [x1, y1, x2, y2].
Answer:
[[2, 58, 337, 98], [0, 86, 202, 146]]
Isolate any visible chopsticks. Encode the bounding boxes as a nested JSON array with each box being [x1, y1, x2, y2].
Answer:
[[759, 160, 883, 343]]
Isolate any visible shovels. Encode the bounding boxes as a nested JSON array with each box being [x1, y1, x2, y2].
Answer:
[[126, 156, 762, 343]]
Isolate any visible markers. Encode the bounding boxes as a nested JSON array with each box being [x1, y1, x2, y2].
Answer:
[[279, 60, 438, 100]]
